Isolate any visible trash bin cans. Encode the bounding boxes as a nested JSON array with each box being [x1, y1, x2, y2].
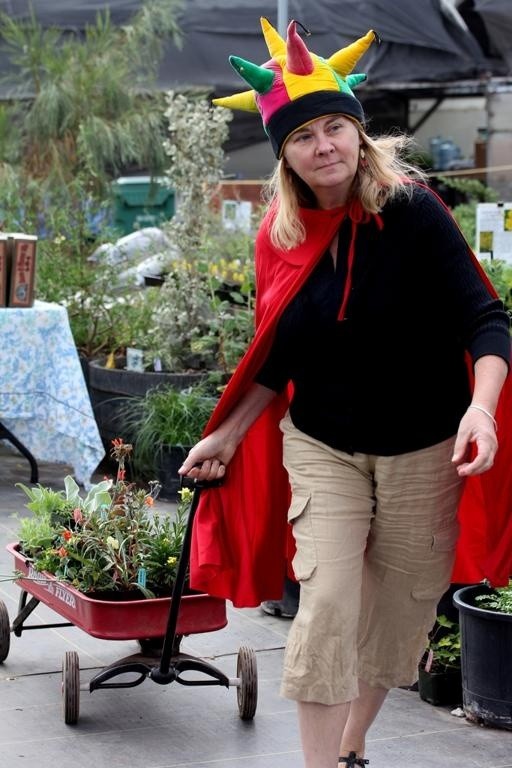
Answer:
[[112, 176, 176, 235]]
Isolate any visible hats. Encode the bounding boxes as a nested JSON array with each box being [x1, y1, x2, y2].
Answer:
[[212, 14, 379, 162]]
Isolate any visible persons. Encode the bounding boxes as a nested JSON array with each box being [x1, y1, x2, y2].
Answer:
[[168, 15, 512, 766]]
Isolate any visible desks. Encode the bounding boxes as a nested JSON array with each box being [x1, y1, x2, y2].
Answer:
[[0, 298, 69, 485]]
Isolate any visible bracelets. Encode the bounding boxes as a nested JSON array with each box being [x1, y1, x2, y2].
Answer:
[[467, 405, 498, 435]]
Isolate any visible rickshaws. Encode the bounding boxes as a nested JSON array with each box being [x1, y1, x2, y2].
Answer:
[[0, 459, 257, 727]]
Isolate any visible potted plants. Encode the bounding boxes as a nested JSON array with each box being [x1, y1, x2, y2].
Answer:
[[95, 382, 217, 501], [416, 579, 511, 733]]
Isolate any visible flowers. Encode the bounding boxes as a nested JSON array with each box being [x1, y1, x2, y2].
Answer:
[[8, 436, 201, 601]]
